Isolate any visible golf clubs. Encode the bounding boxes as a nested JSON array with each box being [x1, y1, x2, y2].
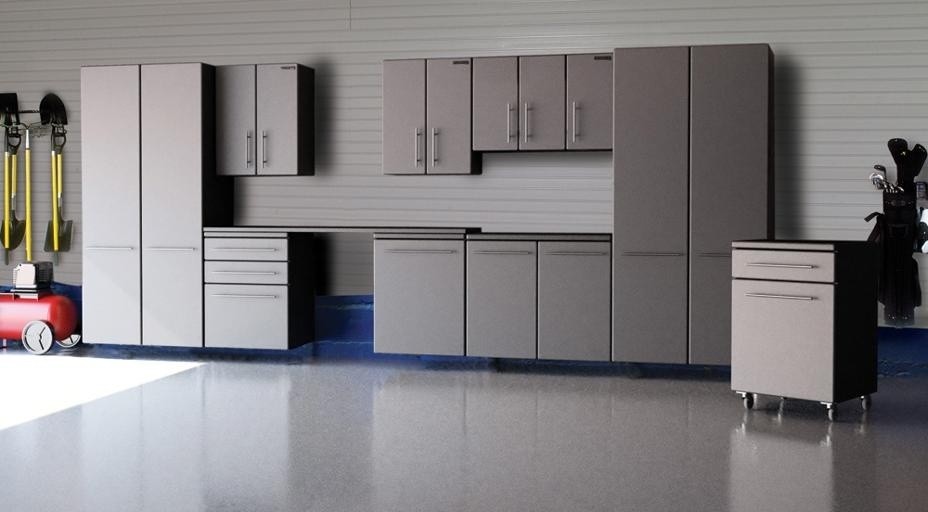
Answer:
[[869, 165, 906, 194]]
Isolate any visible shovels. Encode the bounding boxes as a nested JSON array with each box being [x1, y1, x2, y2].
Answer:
[[0, 93, 75, 266]]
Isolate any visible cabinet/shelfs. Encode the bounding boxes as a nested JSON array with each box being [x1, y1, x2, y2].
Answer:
[[474, 58, 567, 150], [731, 239, 879, 407], [619, 46, 766, 368], [468, 242, 611, 362], [84, 63, 203, 351], [568, 54, 614, 150], [375, 241, 463, 355], [204, 237, 287, 352], [382, 59, 470, 175], [214, 63, 297, 176]]
